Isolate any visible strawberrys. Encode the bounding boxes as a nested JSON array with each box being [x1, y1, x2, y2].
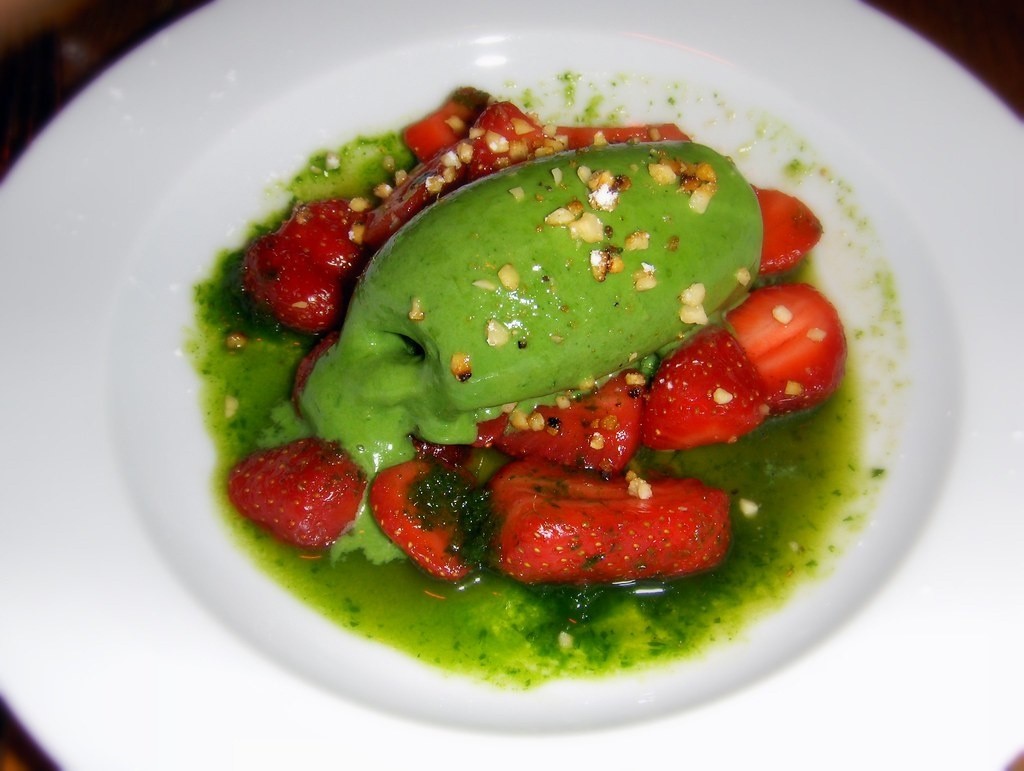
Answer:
[[224, 94, 846, 585]]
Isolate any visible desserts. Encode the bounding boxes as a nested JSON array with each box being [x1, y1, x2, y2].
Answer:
[[293, 141, 765, 448]]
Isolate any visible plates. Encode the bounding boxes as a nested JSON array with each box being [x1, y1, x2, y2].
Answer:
[[0, 1, 1024, 771]]
